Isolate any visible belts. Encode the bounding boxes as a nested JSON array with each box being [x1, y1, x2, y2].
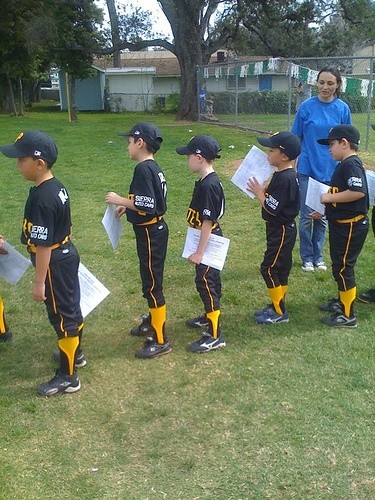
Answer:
[[336, 212, 364, 223], [137, 215, 164, 225], [28, 235, 69, 254]]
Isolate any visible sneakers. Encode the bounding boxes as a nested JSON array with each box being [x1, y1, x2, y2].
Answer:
[[135, 336, 172, 359], [188, 330, 227, 353], [186, 313, 221, 329], [37, 369, 81, 396], [318, 297, 343, 314], [254, 304, 289, 325], [131, 314, 153, 337], [53, 350, 87, 366], [321, 312, 358, 328]]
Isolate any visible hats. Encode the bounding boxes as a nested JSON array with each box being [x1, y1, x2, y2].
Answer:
[[317, 124, 361, 146], [117, 123, 163, 151], [0, 130, 57, 164], [256, 131, 302, 161], [176, 135, 223, 159]]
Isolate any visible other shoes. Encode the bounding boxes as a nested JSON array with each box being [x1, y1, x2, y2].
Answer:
[[315, 261, 327, 270], [357, 288, 375, 304], [301, 261, 315, 272]]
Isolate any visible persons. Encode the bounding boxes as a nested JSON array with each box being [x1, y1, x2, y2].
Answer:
[[206, 94, 215, 113], [290, 64, 353, 271], [0, 130, 87, 396], [307, 124, 369, 329], [105, 122, 173, 358], [246, 131, 300, 324], [199, 80, 207, 113], [0, 235, 13, 340], [174, 135, 226, 352]]
